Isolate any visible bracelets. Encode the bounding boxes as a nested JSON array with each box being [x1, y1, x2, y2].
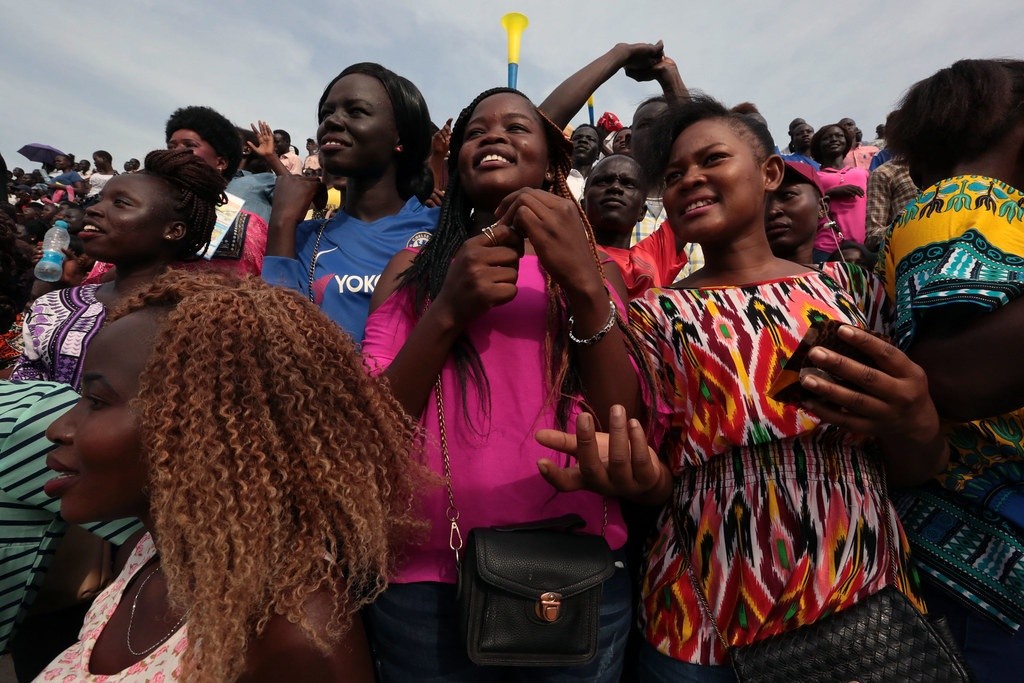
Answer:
[[568, 300, 616, 346]]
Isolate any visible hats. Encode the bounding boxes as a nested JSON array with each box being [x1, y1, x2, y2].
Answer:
[[785, 161, 824, 199], [11, 184, 31, 193]]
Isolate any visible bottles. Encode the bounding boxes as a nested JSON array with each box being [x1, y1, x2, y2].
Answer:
[[34, 220, 71, 282]]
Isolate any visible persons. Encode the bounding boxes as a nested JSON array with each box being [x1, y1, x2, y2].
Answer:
[[30, 270, 435, 683], [0, 42, 1022, 683]]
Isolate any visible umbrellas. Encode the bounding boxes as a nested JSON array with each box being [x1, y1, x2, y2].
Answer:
[[16, 143, 65, 167]]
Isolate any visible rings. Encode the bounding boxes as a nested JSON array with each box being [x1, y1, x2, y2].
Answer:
[[480, 226, 499, 247]]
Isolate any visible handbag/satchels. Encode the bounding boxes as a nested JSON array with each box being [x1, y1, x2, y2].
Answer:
[[729, 586, 973, 683], [45, 522, 114, 615], [457, 514, 615, 670]]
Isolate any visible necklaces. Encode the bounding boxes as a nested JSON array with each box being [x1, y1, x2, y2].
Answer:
[[125, 566, 193, 656]]
